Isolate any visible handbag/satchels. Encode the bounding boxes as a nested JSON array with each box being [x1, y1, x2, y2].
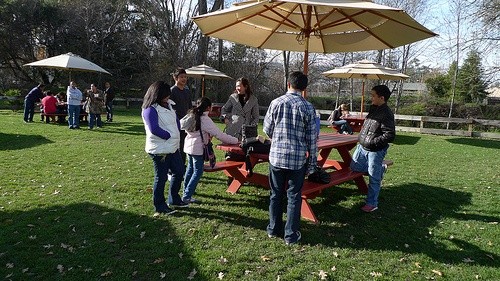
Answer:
[[203, 140, 217, 168], [308, 169, 331, 185], [326, 109, 340, 126]]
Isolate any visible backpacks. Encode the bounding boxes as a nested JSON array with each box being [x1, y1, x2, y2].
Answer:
[[241, 138, 271, 176]]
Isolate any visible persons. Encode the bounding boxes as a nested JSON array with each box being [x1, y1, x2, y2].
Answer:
[[330, 104, 353, 135], [263, 71, 317, 245], [141, 80, 189, 215], [24, 81, 114, 130], [221, 77, 259, 186], [350, 85, 395, 212], [180, 97, 240, 202], [167, 68, 193, 180]]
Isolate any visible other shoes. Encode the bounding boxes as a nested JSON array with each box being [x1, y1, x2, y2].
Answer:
[[268, 232, 278, 238], [182, 197, 198, 204], [361, 205, 378, 212], [381, 164, 388, 180], [156, 207, 176, 215], [286, 231, 302, 246], [167, 200, 188, 207]]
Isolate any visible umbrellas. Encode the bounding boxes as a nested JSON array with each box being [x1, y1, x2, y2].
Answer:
[[189, 0, 437, 98], [185, 62, 233, 97], [322, 59, 410, 133], [23, 52, 111, 83]]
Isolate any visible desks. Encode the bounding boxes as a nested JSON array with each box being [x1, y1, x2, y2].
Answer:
[[61, 103, 84, 123], [217, 133, 367, 223], [341, 116, 368, 133], [212, 106, 223, 122]]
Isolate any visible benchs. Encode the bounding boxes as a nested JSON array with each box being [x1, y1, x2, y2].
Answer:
[[328, 126, 338, 132], [300, 159, 392, 224], [40, 114, 88, 124], [209, 112, 221, 117], [203, 160, 267, 172]]
